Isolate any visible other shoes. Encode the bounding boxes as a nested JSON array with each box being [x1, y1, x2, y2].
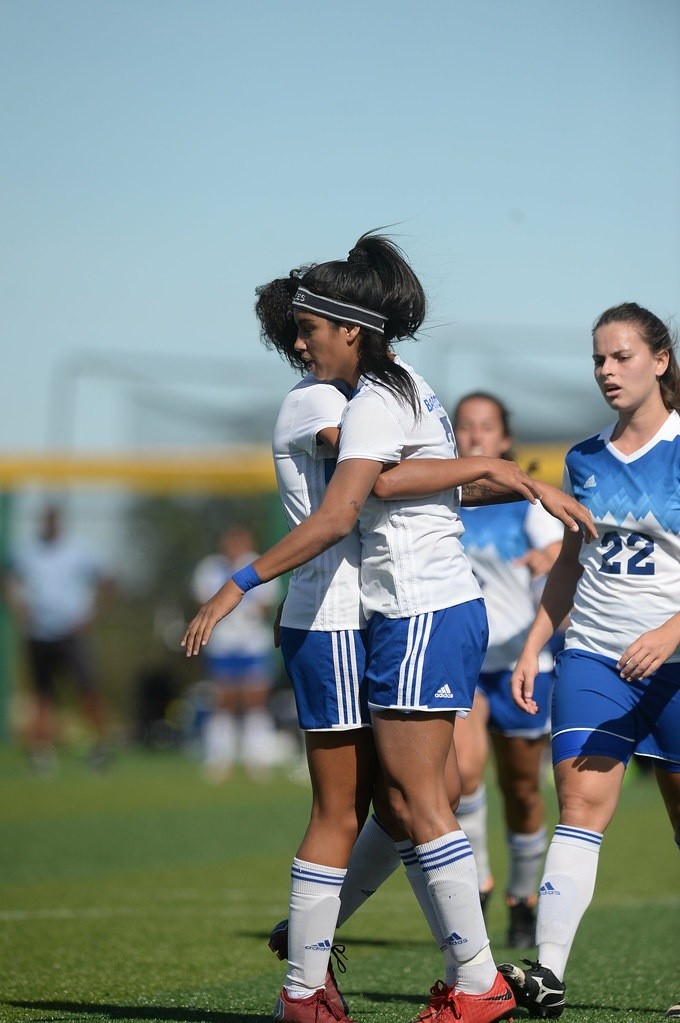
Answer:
[[507, 884, 537, 950], [479, 873, 494, 912]]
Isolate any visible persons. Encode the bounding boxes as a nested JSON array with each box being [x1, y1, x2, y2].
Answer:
[[494, 302, 679, 1018], [0, 221, 598, 1023]]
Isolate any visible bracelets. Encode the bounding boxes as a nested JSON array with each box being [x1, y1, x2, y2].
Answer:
[[232, 563, 263, 593]]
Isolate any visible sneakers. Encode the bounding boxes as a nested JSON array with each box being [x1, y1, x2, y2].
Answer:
[[666, 1002, 680, 1018], [415, 972, 517, 1023], [496, 957, 566, 1019], [274, 988, 358, 1023], [268, 918, 350, 1016]]
